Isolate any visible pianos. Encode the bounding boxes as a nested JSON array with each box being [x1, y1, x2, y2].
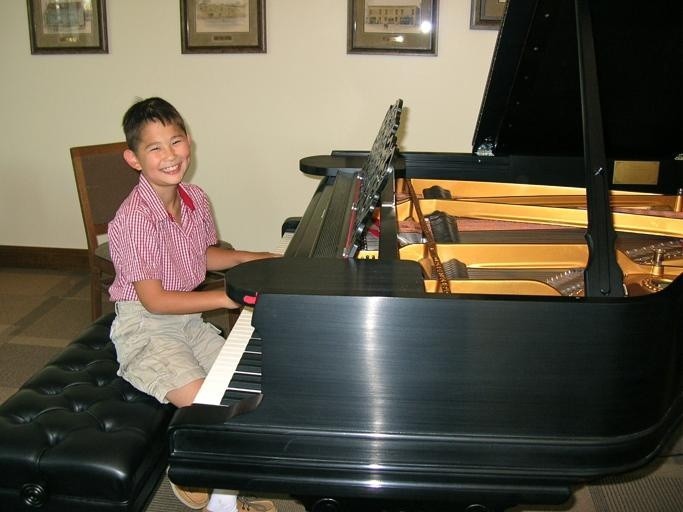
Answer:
[[167, 1, 683, 511]]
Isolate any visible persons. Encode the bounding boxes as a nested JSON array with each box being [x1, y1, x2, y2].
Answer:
[[107, 95, 282, 511]]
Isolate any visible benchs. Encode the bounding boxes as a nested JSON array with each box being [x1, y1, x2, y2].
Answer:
[[0, 314, 178, 511]]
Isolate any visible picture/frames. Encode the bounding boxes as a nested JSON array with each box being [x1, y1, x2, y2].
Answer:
[[345, 1, 439, 56], [470, 1, 509, 29], [26, 0, 105, 57], [179, 1, 265, 56]]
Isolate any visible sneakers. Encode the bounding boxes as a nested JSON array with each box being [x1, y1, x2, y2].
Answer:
[[170, 481, 210, 510], [236, 500, 279, 512]]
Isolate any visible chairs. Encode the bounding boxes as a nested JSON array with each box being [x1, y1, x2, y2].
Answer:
[[70, 142, 240, 331]]
